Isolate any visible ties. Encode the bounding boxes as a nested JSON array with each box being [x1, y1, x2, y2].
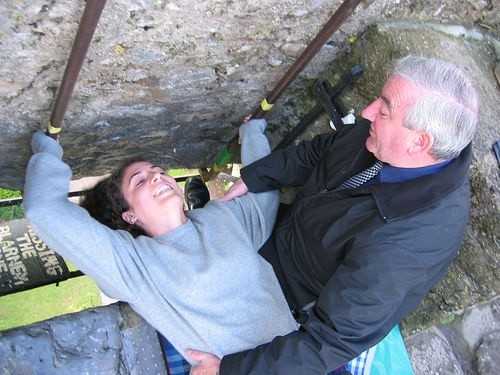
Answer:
[[324, 160, 385, 192]]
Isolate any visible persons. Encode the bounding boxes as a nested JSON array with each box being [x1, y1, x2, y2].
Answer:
[[184, 54, 481, 374], [21, 114, 351, 374]]
